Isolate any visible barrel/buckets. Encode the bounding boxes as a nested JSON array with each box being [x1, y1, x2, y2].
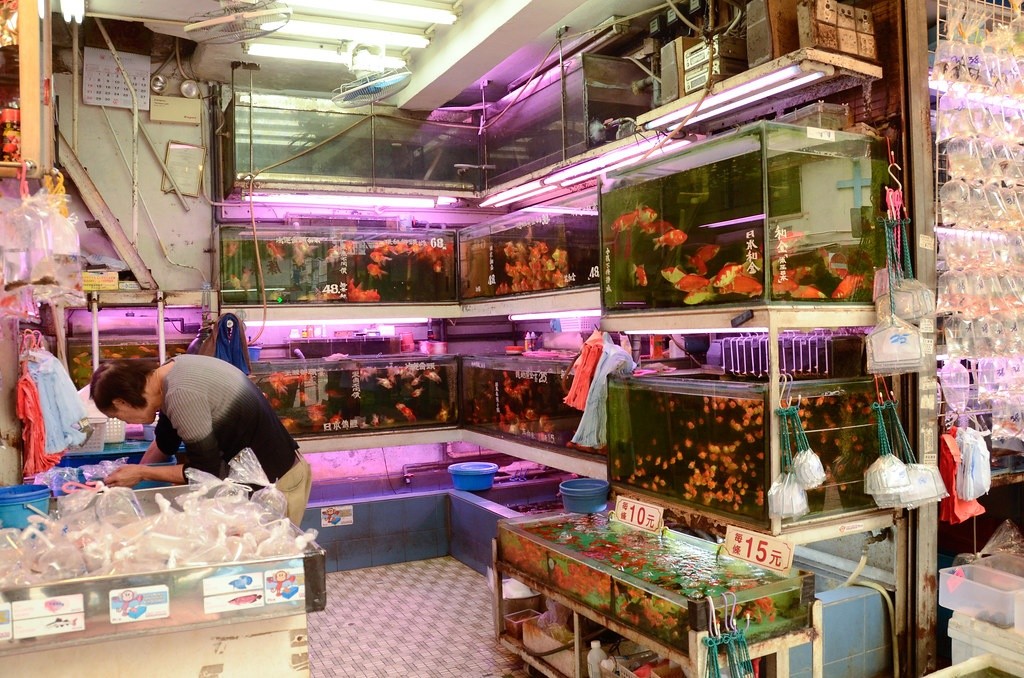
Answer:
[[0, 484, 51, 540]]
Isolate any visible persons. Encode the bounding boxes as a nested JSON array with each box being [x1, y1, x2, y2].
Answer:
[[90, 354, 313, 534]]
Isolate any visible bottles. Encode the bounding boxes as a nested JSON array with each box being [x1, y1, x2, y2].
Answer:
[[523, 331, 537, 352], [587, 641, 607, 678]]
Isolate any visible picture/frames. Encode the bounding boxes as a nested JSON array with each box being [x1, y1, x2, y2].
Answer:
[[161, 139, 207, 198]]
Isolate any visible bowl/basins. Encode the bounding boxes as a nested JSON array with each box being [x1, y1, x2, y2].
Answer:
[[504, 345, 522, 354], [559, 479, 610, 513], [447, 462, 499, 492]]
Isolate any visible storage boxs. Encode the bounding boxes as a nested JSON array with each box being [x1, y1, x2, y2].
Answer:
[[972, 554, 1024, 577], [68, 334, 197, 391], [225, 93, 375, 188], [216, 224, 458, 308], [460, 353, 608, 462], [375, 112, 482, 196], [493, 518, 816, 658], [936, 564, 1024, 627], [244, 353, 459, 440], [456, 205, 603, 305], [947, 611, 1024, 665], [591, 120, 893, 317], [485, 53, 650, 189], [604, 365, 891, 532]]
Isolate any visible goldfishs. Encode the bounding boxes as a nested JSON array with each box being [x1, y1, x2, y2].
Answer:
[[495, 237, 571, 298], [248, 366, 450, 432], [501, 517, 780, 640], [227, 237, 453, 303], [611, 373, 876, 514], [612, 206, 864, 307], [471, 370, 609, 459]]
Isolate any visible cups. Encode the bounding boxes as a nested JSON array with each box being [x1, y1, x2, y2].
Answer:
[[248, 346, 262, 363]]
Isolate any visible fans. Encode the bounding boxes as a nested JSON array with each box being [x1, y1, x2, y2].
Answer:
[[331, 42, 413, 109], [183, 0, 297, 44]]
